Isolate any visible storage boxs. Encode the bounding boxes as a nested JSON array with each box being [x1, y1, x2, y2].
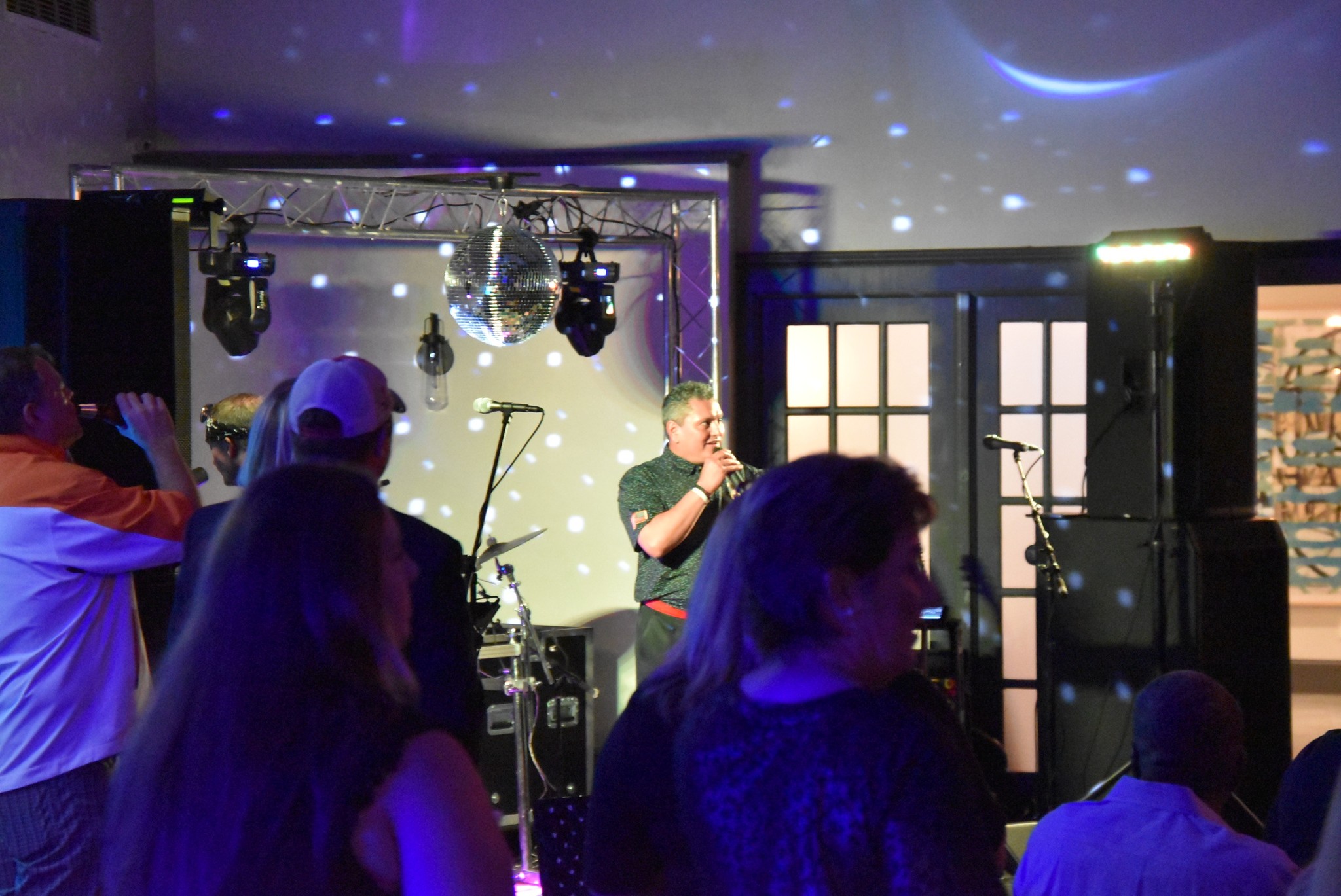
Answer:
[[478, 624, 597, 823]]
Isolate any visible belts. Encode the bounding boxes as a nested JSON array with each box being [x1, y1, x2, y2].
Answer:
[[645, 600, 688, 619]]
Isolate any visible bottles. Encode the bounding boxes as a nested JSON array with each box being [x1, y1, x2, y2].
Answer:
[[77, 402, 125, 428]]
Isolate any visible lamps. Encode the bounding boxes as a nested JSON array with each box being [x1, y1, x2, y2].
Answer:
[[553, 231, 619, 357], [443, 173, 563, 346], [417, 312, 453, 412], [199, 215, 276, 357]]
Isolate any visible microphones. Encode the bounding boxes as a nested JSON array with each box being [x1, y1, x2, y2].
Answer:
[[983, 434, 1039, 452], [473, 397, 544, 414], [714, 447, 746, 495]]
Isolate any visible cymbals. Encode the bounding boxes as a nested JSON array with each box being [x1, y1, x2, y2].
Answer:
[[476, 527, 549, 565]]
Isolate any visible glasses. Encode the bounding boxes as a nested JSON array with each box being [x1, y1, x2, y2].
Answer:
[[198, 403, 217, 424]]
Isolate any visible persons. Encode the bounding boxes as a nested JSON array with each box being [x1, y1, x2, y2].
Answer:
[[1011, 669, 1341, 896], [0, 344, 204, 896], [618, 382, 766, 692], [97, 353, 516, 895], [589, 455, 1007, 895]]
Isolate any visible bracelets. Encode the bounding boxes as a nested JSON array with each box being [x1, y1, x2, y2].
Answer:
[[691, 484, 712, 505]]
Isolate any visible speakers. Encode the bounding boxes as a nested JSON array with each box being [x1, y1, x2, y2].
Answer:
[[1082, 243, 1259, 520], [0, 197, 194, 500], [1031, 513, 1294, 840]]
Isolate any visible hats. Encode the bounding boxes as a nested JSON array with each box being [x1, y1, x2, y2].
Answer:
[[287, 355, 407, 439]]
